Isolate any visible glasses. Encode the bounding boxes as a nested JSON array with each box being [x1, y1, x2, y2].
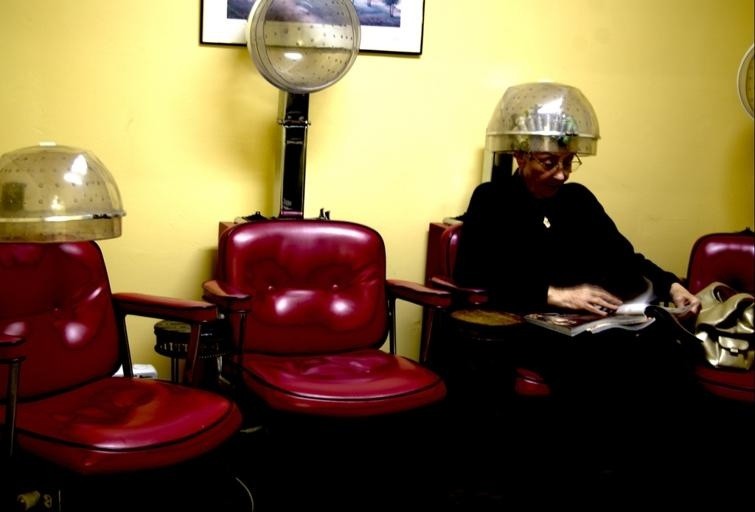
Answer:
[[528, 152, 582, 173]]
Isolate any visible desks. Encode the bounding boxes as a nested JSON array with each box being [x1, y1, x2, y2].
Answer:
[[152, 319, 214, 387], [446, 306, 523, 344]]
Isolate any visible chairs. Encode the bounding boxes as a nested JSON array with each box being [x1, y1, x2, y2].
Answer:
[[192, 219, 453, 419], [419, 221, 553, 398], [3, 229, 256, 512], [683, 227, 754, 401]]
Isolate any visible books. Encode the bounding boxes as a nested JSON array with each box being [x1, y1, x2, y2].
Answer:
[[524, 278, 703, 348]]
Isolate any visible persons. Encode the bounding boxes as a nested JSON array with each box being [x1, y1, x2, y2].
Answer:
[[457, 114, 716, 511]]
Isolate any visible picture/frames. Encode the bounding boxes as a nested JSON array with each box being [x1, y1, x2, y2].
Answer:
[[199, 0, 425, 56]]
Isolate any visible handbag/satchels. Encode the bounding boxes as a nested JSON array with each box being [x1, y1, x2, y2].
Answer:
[[694, 283, 755, 371]]
